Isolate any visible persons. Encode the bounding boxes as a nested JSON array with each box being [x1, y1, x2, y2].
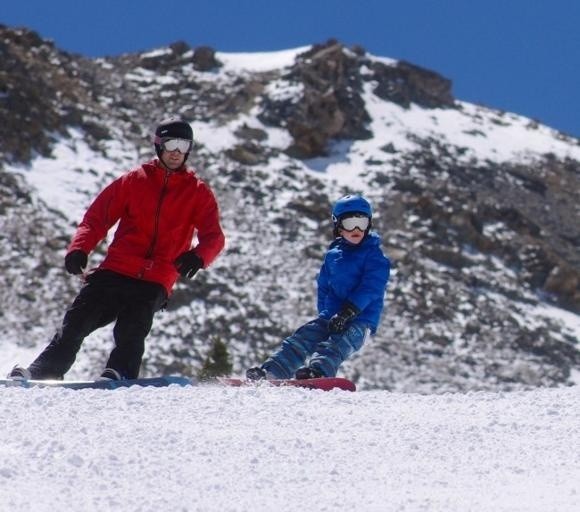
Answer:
[[8, 118, 226, 382], [245, 193, 391, 381]]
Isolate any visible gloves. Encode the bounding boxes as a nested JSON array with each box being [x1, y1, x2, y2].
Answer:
[[66, 250, 88, 274], [329, 301, 360, 334], [175, 250, 203, 278]]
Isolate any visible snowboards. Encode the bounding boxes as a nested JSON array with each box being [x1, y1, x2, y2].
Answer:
[[0, 376, 189, 388], [218, 377, 356, 392]]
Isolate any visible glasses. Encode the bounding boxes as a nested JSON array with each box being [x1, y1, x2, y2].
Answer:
[[332, 214, 370, 232], [155, 135, 192, 154]]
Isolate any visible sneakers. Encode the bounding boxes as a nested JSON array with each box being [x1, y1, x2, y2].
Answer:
[[11, 365, 34, 378], [247, 368, 272, 382], [99, 368, 122, 383], [295, 367, 321, 382]]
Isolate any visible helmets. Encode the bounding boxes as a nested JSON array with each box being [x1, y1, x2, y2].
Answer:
[[332, 194, 373, 221], [155, 118, 193, 160]]
